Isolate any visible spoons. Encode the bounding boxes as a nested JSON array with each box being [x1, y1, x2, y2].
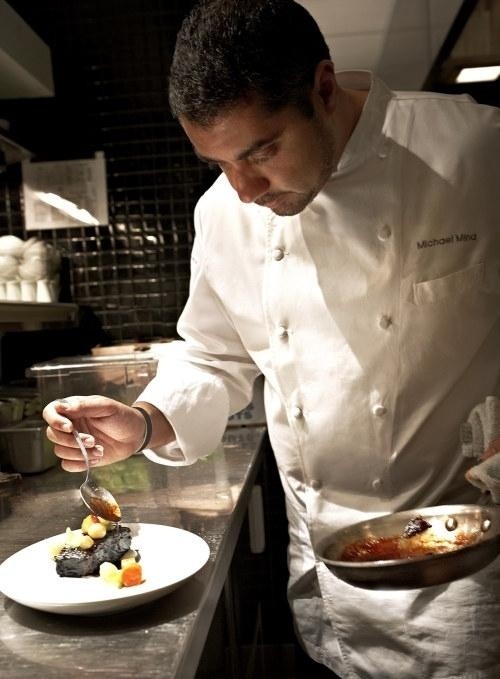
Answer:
[[68, 427, 122, 523]]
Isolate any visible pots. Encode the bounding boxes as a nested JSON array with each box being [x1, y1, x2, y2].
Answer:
[[315, 489, 500, 589]]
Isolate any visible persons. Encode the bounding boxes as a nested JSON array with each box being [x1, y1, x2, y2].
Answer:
[[43, 0, 500, 679]]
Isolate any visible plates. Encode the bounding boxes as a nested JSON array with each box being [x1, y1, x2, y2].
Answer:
[[0, 522, 211, 618]]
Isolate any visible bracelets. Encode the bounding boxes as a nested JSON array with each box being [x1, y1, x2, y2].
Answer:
[[132, 407, 152, 453]]
